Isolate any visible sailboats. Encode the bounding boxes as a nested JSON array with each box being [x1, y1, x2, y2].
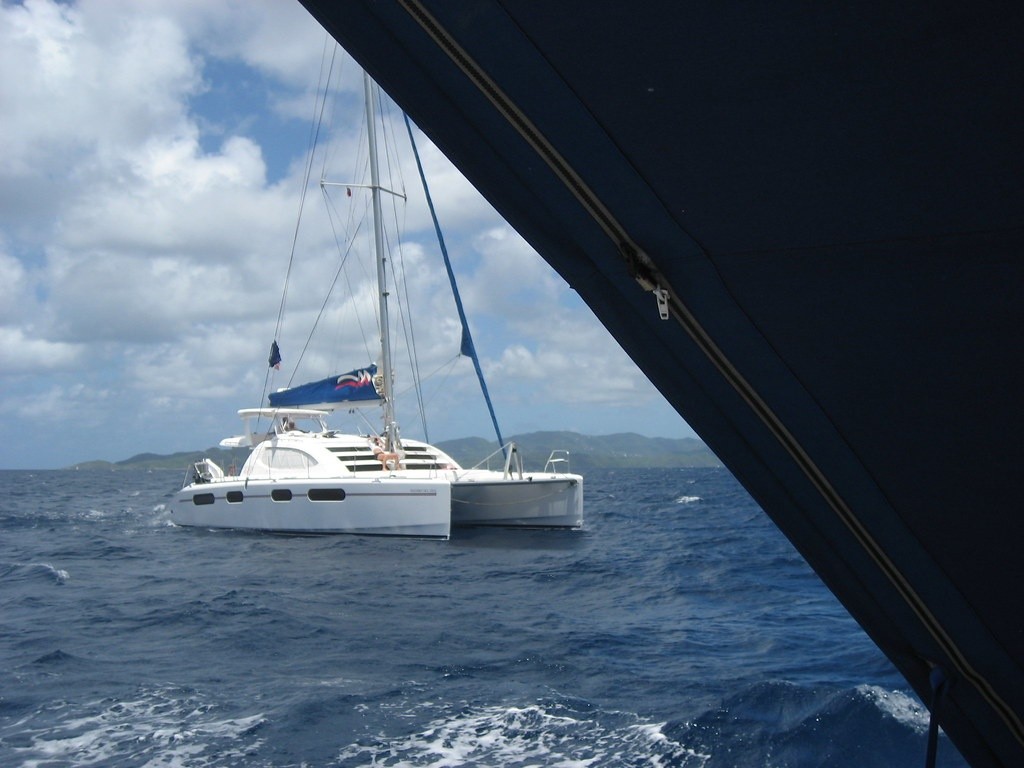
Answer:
[[174, 29, 582, 535]]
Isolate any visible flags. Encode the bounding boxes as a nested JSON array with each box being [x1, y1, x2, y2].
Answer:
[[268, 340, 281, 370]]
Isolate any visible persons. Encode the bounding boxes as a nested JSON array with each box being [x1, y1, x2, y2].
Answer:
[[366, 435, 400, 471], [283, 418, 300, 432]]
